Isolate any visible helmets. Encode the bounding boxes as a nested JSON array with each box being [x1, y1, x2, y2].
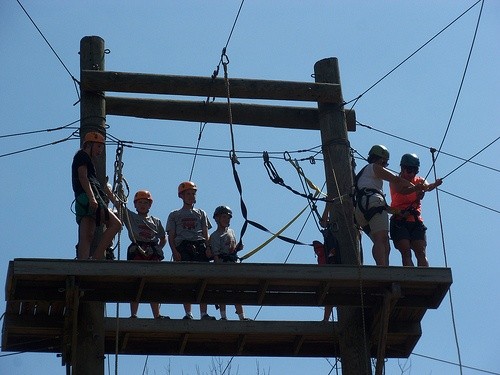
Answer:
[[134, 190, 153, 201], [213, 206, 232, 218], [368, 145, 389, 159], [178, 182, 197, 193], [83, 131, 107, 145], [400, 154, 420, 167]]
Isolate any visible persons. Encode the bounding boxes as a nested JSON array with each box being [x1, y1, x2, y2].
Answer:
[[211, 205, 253, 321], [102, 174, 171, 319], [354, 145, 421, 266], [318, 200, 340, 321], [388, 153, 443, 267], [166, 181, 216, 320], [70, 130, 122, 261]]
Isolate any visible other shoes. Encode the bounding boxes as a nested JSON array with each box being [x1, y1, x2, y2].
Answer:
[[201, 314, 216, 320], [154, 315, 170, 320], [182, 314, 193, 320], [219, 318, 227, 320], [240, 317, 252, 321], [129, 315, 137, 318]]
